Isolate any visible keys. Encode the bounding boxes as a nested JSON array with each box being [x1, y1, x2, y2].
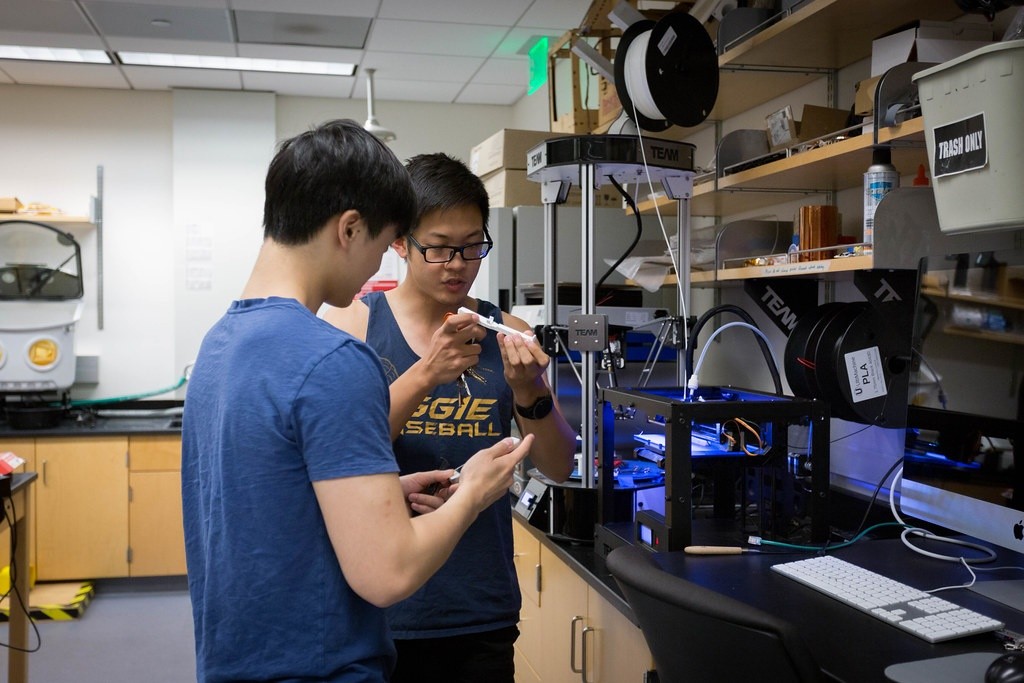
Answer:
[[456, 364, 493, 407]]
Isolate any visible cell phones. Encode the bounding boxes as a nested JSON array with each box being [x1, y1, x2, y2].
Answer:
[[449, 437, 520, 484]]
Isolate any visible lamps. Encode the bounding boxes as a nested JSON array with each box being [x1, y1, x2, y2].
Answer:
[[361, 68, 397, 143]]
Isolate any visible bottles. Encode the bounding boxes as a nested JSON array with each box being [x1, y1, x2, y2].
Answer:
[[863, 140, 901, 246]]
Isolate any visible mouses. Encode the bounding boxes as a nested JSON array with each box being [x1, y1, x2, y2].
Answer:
[[984, 649, 1024, 683]]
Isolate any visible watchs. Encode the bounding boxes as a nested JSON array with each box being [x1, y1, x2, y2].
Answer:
[[514, 386, 553, 420]]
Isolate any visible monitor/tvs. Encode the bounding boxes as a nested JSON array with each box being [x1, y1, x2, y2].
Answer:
[[897, 246, 1024, 613]]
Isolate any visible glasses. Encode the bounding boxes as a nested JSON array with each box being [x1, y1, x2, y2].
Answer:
[[405, 224, 493, 263]]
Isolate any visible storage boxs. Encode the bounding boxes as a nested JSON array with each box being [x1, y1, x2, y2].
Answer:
[[854, 74, 884, 116], [469, 129, 587, 179], [598, 78, 623, 126], [910, 39, 1024, 237], [482, 167, 623, 208], [764, 103, 851, 158], [871, 19, 997, 78]]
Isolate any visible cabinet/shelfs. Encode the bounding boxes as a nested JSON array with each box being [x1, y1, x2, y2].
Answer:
[[0, 434, 129, 581], [128, 435, 189, 577], [625, 0, 1009, 343], [513, 517, 655, 683]]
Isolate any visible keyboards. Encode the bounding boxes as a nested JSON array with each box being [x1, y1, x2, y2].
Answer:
[[770, 556, 1005, 643]]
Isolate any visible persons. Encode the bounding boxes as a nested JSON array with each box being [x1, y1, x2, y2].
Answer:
[[323, 153, 576, 683], [179, 119, 534, 683]]
